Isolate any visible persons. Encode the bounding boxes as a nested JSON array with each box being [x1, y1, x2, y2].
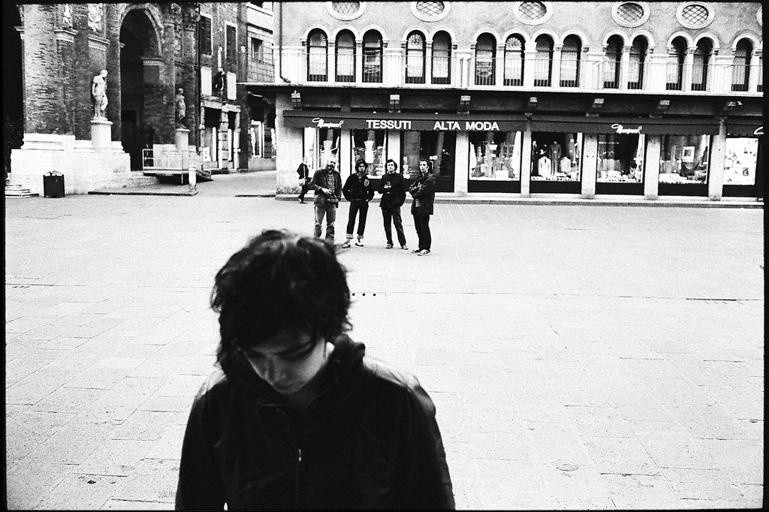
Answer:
[[408, 159, 435, 257], [376, 159, 409, 249], [296, 156, 312, 203], [309, 158, 342, 242], [169, 225, 456, 510], [175, 88, 187, 129], [341, 160, 374, 248], [548, 142, 562, 174], [91, 70, 110, 118]]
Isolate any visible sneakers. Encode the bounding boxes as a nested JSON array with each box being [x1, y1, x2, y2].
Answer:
[[386, 243, 409, 250], [342, 240, 363, 248], [298, 196, 307, 204], [411, 246, 430, 256]]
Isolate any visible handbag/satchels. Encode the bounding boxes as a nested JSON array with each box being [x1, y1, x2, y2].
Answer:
[[299, 178, 306, 186]]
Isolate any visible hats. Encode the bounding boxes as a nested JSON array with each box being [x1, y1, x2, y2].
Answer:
[[355, 159, 368, 171]]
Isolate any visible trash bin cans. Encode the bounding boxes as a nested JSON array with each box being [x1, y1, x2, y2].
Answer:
[[43, 170, 65, 198]]
